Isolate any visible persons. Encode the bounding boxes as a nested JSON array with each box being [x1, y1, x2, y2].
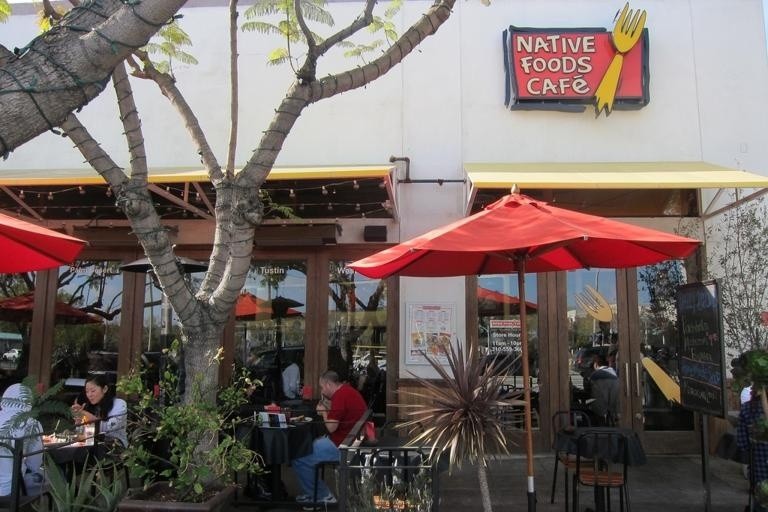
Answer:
[[288, 367, 370, 512], [734, 380, 768, 512], [283, 350, 306, 400], [593, 355, 619, 380], [68, 373, 130, 476], [0, 382, 52, 511]]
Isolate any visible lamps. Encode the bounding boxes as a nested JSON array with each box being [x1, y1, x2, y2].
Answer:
[[121, 255, 209, 406]]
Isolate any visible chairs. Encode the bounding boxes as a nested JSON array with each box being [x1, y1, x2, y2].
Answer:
[[575, 432, 629, 512], [91, 432, 129, 497], [314, 462, 353, 511], [551, 410, 603, 504], [0, 443, 55, 512]]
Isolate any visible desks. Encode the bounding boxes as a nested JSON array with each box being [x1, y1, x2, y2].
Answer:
[[553, 426, 645, 512]]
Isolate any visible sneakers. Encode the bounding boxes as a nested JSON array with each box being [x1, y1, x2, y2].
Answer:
[[296, 494, 339, 510]]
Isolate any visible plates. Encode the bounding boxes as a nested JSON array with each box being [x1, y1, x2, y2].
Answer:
[[291, 417, 312, 424], [43, 439, 70, 446]]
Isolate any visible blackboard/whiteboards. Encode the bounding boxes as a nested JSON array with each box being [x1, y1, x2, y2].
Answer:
[[676, 278, 727, 419]]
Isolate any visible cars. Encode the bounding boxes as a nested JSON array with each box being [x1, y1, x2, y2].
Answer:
[[247, 346, 344, 374], [2, 349, 22, 363], [53, 351, 149, 393], [354, 352, 386, 371], [478, 352, 584, 392]]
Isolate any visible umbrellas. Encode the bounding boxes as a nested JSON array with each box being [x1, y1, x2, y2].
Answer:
[[476, 286, 540, 318], [346, 184, 703, 492], [0, 213, 94, 276], [231, 290, 308, 320], [0, 288, 104, 329]]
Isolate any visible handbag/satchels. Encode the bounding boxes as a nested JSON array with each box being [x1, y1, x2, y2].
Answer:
[[242, 479, 289, 503]]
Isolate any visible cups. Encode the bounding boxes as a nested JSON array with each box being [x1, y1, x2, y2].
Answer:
[[85, 428, 95, 445]]
